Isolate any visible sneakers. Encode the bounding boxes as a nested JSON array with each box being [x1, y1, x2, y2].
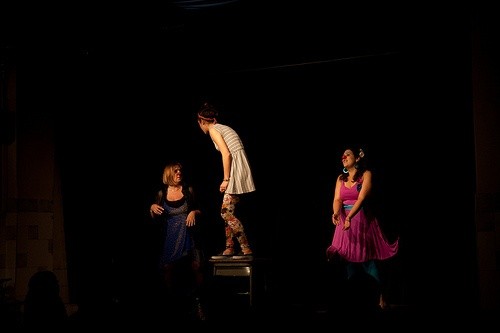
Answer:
[[211, 251, 236, 259], [231, 249, 254, 259]]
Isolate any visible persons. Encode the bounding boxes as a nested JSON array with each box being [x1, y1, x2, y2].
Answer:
[[327, 149, 397, 312], [150, 163, 205, 320], [197, 108, 255, 261]]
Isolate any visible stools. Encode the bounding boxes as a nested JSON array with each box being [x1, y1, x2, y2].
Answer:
[[209, 256, 255, 305]]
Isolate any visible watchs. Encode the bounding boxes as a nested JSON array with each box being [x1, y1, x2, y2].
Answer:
[[224, 177, 230, 181]]
